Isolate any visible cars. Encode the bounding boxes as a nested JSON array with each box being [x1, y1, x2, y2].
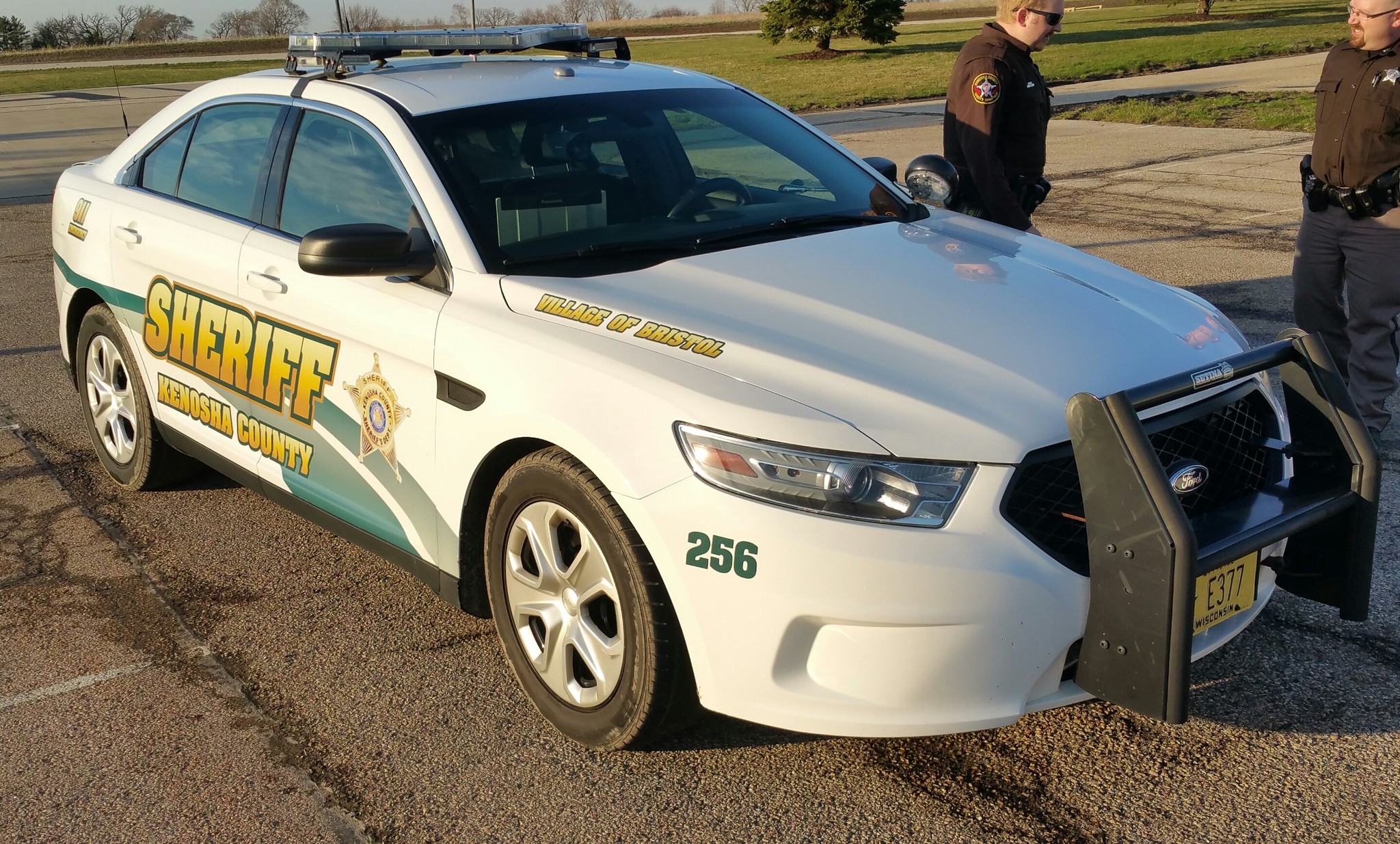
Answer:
[[44, 18, 1384, 755]]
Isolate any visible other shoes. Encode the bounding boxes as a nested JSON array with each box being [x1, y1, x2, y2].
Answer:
[[1367, 426, 1382, 453]]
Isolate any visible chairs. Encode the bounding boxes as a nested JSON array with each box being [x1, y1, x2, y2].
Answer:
[[498, 116, 634, 224]]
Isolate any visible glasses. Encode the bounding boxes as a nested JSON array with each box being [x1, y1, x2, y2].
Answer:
[[1346, 3, 1400, 21], [1012, 7, 1064, 26]]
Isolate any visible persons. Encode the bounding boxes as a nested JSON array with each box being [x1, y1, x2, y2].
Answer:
[[944, 0, 1065, 235], [1291, 0, 1400, 451]]
